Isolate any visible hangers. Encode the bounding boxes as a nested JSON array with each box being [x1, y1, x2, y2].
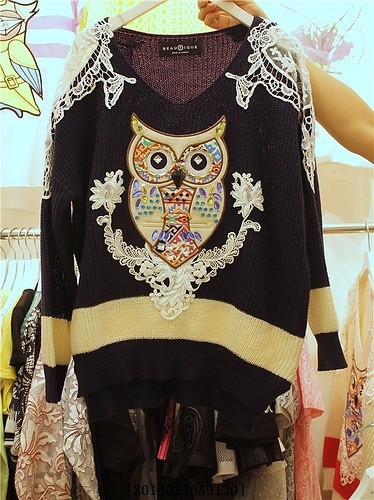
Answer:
[[0, 224, 49, 291], [91, 0, 258, 47], [353, 219, 374, 289]]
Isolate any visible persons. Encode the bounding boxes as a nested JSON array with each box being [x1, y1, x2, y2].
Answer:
[[194, 0, 374, 165]]
